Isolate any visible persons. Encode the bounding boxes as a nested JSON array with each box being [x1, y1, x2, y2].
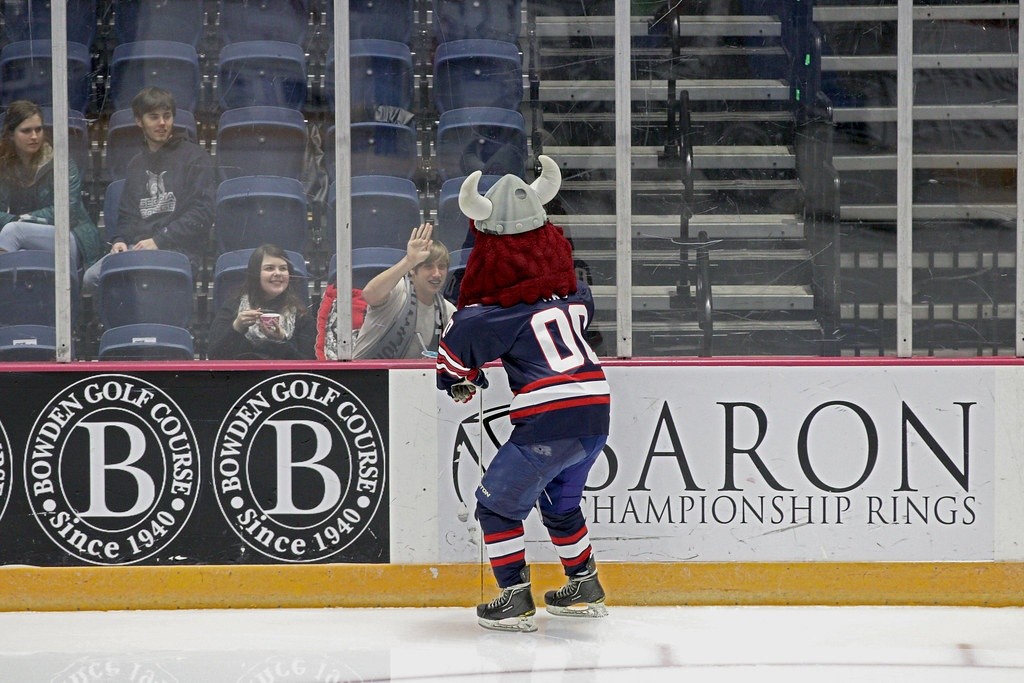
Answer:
[[0, 100, 101, 270], [352, 223, 457, 360], [82, 85, 217, 334], [435, 156, 610, 633], [207, 244, 317, 361]]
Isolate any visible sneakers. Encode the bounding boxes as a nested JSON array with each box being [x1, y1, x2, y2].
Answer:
[[545, 553, 607, 617], [477, 565, 537, 632]]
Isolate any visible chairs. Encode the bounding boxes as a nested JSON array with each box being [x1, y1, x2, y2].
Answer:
[[0, 0, 541, 368]]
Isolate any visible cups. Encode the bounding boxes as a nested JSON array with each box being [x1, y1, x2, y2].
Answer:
[[260, 313, 279, 332]]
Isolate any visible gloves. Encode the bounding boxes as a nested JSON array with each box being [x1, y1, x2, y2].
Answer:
[[449, 371, 489, 403]]
